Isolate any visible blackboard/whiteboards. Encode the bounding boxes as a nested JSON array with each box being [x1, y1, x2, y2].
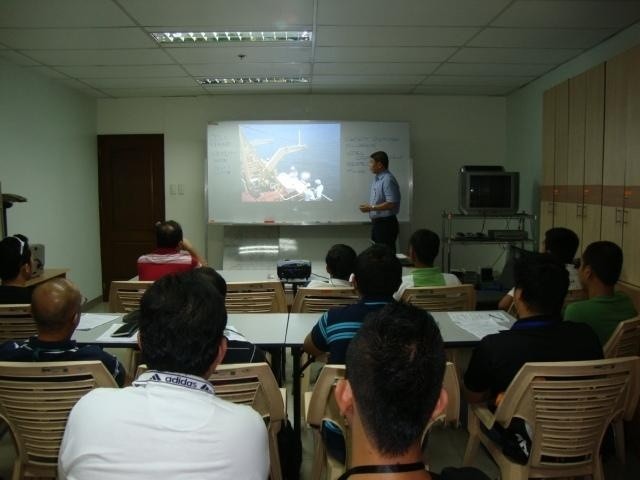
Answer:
[[206, 120, 413, 227]]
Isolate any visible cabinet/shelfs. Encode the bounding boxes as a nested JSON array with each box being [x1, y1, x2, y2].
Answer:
[[442, 209, 533, 272], [540, 50, 640, 290]]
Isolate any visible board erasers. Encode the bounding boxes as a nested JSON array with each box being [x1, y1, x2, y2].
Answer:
[[264, 221, 275, 224]]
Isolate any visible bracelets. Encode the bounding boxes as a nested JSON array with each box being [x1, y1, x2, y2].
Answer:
[[371, 205, 374, 210]]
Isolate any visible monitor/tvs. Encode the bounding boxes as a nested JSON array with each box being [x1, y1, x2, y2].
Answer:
[[459, 166, 520, 215]]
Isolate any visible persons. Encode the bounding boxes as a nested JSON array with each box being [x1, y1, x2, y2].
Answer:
[[393, 228, 463, 302], [464, 250, 605, 469], [305, 244, 358, 300], [193, 266, 294, 438], [334, 302, 490, 480], [0, 277, 130, 429], [303, 244, 404, 366], [57, 270, 272, 479], [0, 233, 37, 320], [561, 240, 637, 346], [137, 219, 206, 294], [283, 166, 323, 201], [359, 151, 401, 254], [496, 226, 583, 311]]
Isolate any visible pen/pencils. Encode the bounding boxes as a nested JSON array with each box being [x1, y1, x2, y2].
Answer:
[[489, 313, 505, 321]]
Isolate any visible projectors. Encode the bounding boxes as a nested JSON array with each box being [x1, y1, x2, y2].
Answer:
[[276, 259, 312, 280]]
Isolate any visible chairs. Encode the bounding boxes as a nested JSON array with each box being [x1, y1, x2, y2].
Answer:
[[0, 280, 639, 478]]
